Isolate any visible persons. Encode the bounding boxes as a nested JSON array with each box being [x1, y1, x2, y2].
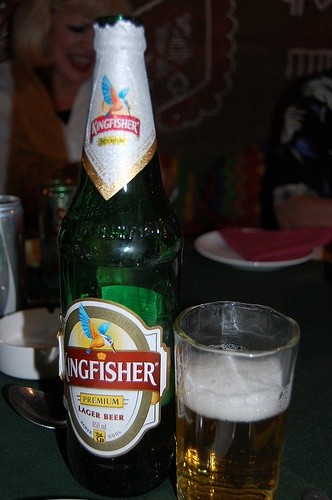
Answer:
[[0, 0, 132, 205], [271, 67, 331, 228]]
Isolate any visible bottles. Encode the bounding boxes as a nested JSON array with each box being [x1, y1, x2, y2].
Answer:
[[56, 14, 185, 497]]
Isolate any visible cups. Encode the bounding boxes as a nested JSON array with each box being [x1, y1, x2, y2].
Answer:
[[173, 301, 299, 500]]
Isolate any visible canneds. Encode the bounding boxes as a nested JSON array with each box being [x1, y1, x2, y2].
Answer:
[[37, 187, 77, 284], [0, 194, 25, 317]]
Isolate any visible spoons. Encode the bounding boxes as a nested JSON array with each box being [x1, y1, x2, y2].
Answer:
[[9, 384, 66, 429]]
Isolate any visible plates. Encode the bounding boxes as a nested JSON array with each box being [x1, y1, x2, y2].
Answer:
[[0, 309, 58, 381], [194, 227, 316, 270]]
